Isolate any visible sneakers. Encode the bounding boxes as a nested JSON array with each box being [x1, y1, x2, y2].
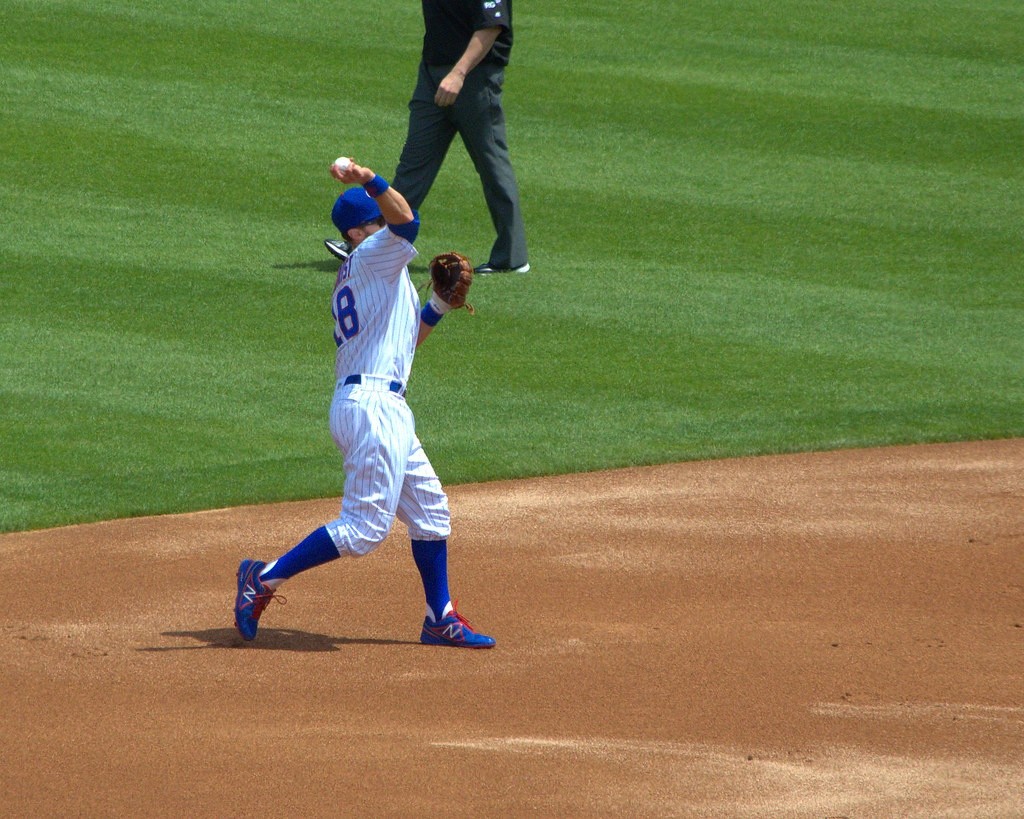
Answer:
[[420, 598, 496, 647], [235, 559, 288, 640], [474, 261, 530, 275], [324, 239, 352, 262]]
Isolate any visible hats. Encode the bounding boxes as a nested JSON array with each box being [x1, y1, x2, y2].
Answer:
[[332, 188, 382, 229]]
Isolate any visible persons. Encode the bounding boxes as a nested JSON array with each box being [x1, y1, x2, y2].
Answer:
[[324, 0, 530, 276], [233, 157, 496, 650]]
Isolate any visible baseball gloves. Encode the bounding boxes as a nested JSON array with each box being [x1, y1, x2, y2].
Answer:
[[430, 253, 473, 308]]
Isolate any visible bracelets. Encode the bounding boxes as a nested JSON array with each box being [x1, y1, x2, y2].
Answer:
[[360, 174, 391, 200]]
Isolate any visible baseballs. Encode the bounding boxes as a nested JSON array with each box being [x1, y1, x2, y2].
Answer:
[[334, 156, 351, 174]]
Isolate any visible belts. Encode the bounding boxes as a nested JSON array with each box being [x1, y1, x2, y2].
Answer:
[[344, 374, 407, 397]]
